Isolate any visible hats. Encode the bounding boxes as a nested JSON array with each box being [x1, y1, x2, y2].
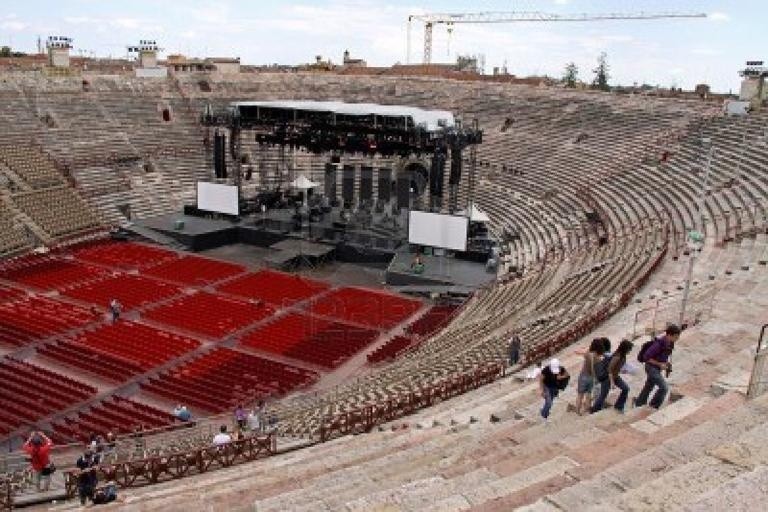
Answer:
[[550, 358, 560, 374]]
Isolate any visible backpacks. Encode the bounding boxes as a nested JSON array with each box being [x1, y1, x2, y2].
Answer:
[[638, 337, 674, 362]]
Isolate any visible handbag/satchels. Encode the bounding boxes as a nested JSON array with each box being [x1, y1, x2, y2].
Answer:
[[595, 353, 616, 381]]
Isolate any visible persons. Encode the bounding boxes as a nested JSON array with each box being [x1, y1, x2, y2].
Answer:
[[634, 324, 681, 413], [174, 404, 182, 423], [21, 429, 53, 491], [110, 297, 123, 322], [591, 339, 635, 415], [74, 449, 107, 511], [573, 338, 605, 416], [539, 357, 571, 426], [178, 406, 191, 423], [211, 398, 279, 448], [592, 337, 614, 408], [508, 334, 524, 367], [89, 430, 116, 464]]
